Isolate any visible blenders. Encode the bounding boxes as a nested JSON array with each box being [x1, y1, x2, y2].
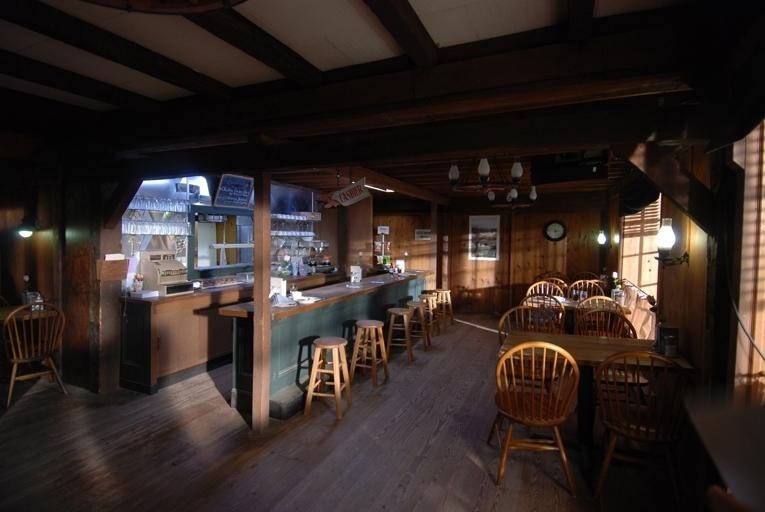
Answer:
[[297, 239, 334, 272]]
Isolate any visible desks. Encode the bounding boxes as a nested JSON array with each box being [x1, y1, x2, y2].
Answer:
[[486, 332, 689, 447]]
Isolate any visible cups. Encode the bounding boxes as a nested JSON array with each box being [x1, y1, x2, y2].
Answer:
[[121, 218, 192, 236], [126, 197, 190, 210]]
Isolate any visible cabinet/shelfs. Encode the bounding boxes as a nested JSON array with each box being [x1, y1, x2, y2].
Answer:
[[122, 202, 192, 290], [269, 212, 321, 271]]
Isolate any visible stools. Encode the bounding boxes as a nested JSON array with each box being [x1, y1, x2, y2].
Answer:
[[415, 294, 440, 336], [401, 302, 430, 352], [424, 289, 453, 335], [386, 308, 414, 363], [349, 319, 389, 386], [304, 336, 351, 420]]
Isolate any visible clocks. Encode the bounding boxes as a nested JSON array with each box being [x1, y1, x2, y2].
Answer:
[[545, 221, 566, 241]]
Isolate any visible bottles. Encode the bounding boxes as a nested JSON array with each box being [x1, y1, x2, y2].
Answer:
[[618, 292, 625, 306]]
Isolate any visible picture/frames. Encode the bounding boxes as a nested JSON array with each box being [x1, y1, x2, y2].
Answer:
[[468, 215, 500, 261]]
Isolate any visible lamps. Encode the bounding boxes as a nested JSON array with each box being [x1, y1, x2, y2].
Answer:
[[449, 158, 524, 193], [16, 217, 33, 239], [487, 185, 537, 210], [654, 217, 689, 266], [597, 226, 611, 253]]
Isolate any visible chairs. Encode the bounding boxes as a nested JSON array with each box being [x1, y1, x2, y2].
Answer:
[[2, 302, 68, 409], [498, 271, 638, 338], [594, 352, 688, 489], [497, 340, 581, 498]]
